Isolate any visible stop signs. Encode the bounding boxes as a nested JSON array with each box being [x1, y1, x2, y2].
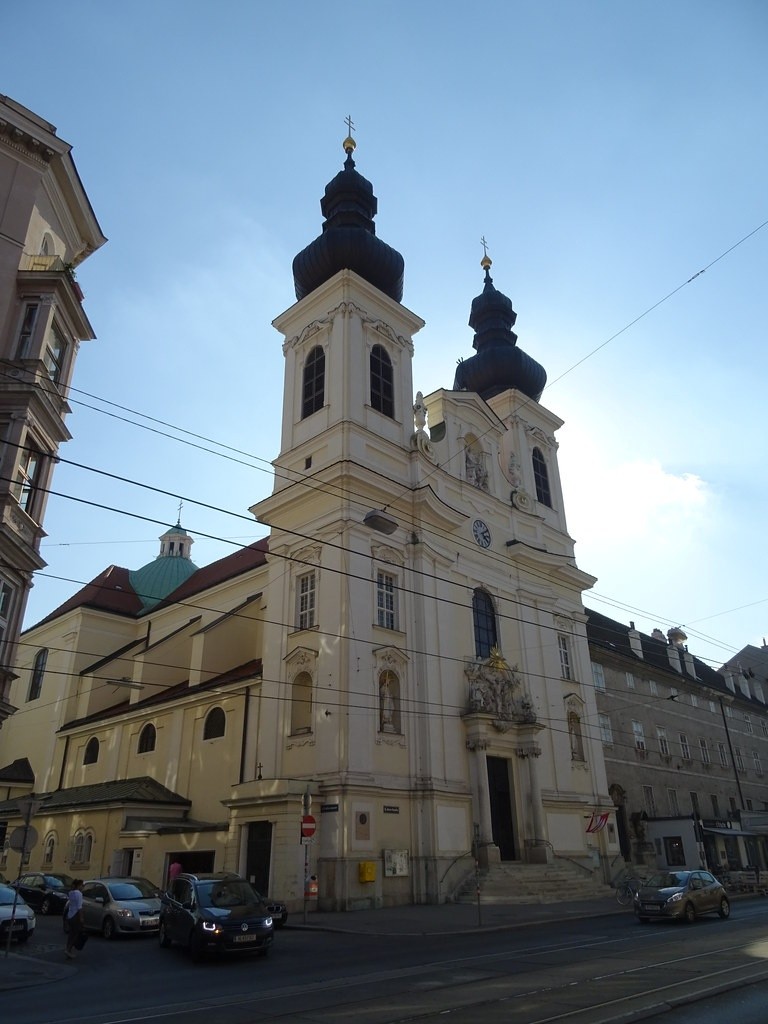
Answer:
[[299, 814, 317, 837]]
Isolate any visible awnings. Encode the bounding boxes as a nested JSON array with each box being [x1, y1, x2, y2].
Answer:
[[703, 828, 758, 837]]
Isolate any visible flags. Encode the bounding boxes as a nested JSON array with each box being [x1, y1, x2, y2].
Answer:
[[586, 812, 610, 834]]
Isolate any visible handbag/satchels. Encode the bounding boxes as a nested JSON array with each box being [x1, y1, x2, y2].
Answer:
[[75, 928, 89, 951]]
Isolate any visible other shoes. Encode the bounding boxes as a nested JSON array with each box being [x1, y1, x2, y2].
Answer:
[[64, 950, 77, 958]]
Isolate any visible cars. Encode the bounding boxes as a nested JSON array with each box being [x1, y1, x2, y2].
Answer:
[[10, 872, 82, 916], [61, 874, 164, 941], [0, 882, 36, 945], [633, 868, 731, 925], [252, 886, 288, 929]]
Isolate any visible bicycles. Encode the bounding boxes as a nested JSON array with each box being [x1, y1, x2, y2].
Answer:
[[615, 867, 661, 907]]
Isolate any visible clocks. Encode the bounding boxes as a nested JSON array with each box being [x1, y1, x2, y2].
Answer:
[[473, 520, 491, 548]]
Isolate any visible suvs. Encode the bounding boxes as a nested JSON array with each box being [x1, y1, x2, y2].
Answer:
[[159, 871, 275, 963]]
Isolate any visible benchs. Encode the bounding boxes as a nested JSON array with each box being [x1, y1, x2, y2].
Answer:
[[722, 871, 768, 896]]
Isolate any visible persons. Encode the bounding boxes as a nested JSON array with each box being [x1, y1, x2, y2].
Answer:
[[168, 859, 182, 896], [486, 676, 507, 720], [63, 878, 85, 958]]
